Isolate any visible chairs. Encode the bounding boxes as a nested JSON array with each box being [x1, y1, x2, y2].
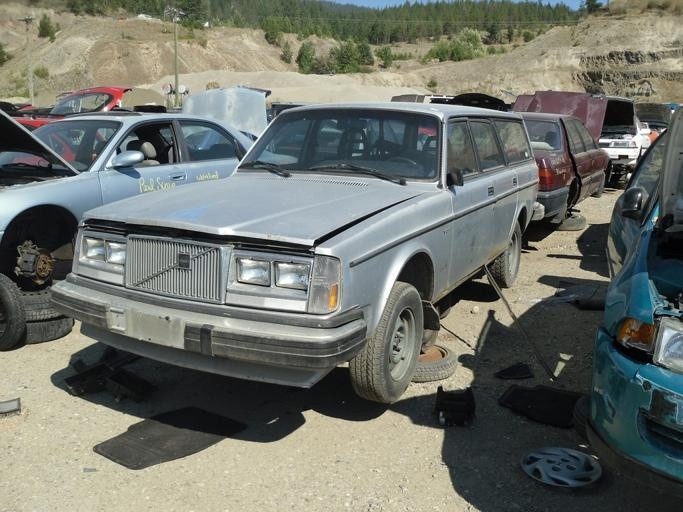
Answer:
[[95, 137, 233, 167], [334, 128, 453, 176]]
